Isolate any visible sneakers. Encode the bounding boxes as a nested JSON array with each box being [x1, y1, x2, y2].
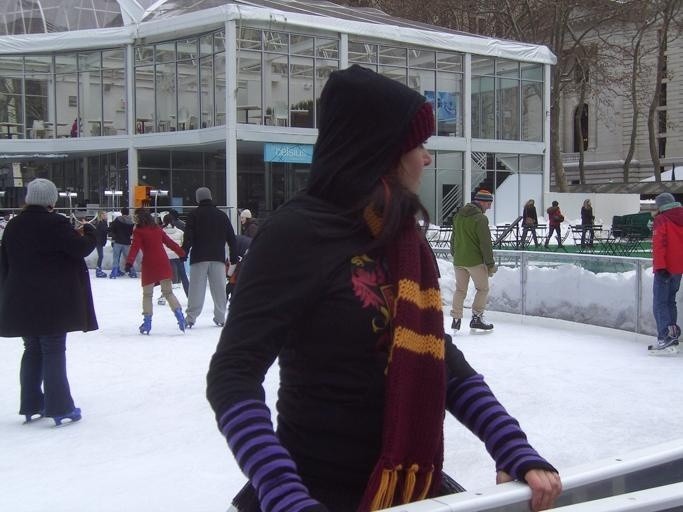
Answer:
[[471, 318, 495, 329], [55, 407, 81, 424], [96, 267, 138, 277], [24, 410, 48, 421], [450, 317, 460, 329], [647, 324, 679, 350]]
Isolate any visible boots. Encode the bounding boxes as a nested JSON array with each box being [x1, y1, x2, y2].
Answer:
[[175, 309, 187, 329], [140, 316, 151, 330]]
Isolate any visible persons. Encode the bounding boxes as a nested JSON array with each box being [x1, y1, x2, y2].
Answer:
[[0, 206, 260, 312], [544, 200, 566, 250], [0, 176, 100, 426], [181, 184, 240, 328], [123, 208, 190, 335], [201, 59, 566, 512], [647, 190, 683, 351], [447, 187, 500, 335], [580, 197, 596, 249], [70, 117, 84, 137], [515, 199, 541, 250]]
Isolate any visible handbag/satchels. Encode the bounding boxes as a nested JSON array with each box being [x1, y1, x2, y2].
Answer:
[[553, 214, 564, 221], [525, 217, 534, 224]]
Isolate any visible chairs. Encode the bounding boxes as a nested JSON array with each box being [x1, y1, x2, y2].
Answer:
[[426, 218, 654, 260]]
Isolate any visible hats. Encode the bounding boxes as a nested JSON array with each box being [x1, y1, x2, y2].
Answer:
[[383, 97, 434, 166], [196, 187, 212, 203], [26, 178, 59, 207], [655, 192, 674, 210], [239, 209, 252, 217], [474, 190, 492, 202]]
